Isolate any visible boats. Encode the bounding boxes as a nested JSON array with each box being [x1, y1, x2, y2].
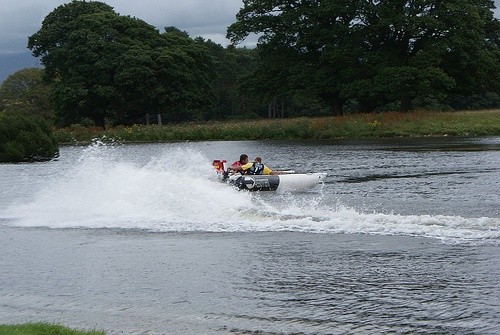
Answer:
[[207, 159, 328, 194]]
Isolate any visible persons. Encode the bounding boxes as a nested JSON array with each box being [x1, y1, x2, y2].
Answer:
[[230, 154, 249, 175], [228, 155, 287, 176]]
